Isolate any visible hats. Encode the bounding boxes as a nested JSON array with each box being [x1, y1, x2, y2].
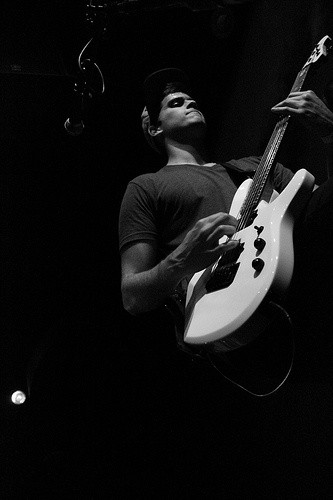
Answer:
[[141, 67, 186, 156]]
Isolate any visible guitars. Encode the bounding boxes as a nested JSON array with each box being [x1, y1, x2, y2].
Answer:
[[182, 34, 331, 345]]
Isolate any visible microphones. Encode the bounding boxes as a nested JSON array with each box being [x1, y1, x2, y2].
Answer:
[[63, 75, 87, 136]]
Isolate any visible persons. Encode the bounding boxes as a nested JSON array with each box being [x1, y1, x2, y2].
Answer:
[[119, 68, 333, 500]]
